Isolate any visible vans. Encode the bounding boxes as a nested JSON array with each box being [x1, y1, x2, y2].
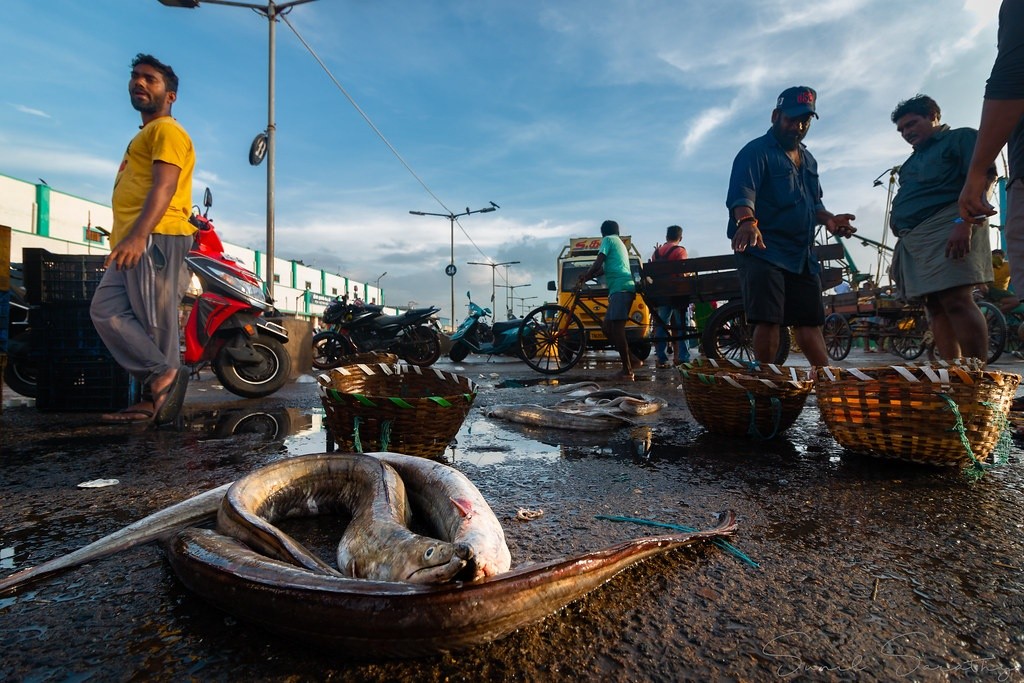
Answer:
[[555, 243, 654, 364]]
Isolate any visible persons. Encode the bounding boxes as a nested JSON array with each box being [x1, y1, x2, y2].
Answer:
[[577, 221, 643, 377], [726, 86, 858, 366], [645, 226, 718, 368], [891, 95, 998, 372], [958, 0, 1024, 436], [978, 250, 1019, 329], [507, 309, 517, 320], [96, 53, 195, 424], [863, 282, 889, 353]]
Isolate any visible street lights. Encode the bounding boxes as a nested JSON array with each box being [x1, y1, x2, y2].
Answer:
[[507, 296, 539, 318], [466, 260, 522, 328], [494, 284, 531, 319], [409, 206, 497, 333]]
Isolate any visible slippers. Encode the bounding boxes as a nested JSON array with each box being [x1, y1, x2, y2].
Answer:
[[106, 409, 152, 424], [151, 366, 191, 425]]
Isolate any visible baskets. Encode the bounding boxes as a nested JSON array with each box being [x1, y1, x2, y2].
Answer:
[[318, 363, 480, 457], [810, 365, 1022, 464], [891, 356, 984, 372], [682, 357, 815, 436]]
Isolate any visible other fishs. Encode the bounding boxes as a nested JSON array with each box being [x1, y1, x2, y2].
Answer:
[[484, 381, 668, 432], [1, 451, 737, 660]]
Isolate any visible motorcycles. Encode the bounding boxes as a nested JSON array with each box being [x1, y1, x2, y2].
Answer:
[[310, 287, 443, 369]]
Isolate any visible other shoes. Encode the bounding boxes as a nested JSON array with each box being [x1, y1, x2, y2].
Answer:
[[631, 361, 644, 369], [673, 359, 686, 366], [656, 361, 670, 368], [610, 372, 634, 379]]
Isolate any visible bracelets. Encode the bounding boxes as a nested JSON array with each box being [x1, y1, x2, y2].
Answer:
[[736, 216, 758, 228]]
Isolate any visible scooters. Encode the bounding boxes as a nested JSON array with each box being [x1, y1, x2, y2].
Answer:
[[451, 289, 539, 365], [1, 187, 292, 403]]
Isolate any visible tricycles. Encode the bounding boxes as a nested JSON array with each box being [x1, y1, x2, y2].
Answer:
[[517, 242, 847, 374], [820, 285, 1024, 368]]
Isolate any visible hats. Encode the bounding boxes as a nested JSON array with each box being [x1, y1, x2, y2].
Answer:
[[778, 85, 818, 119]]
